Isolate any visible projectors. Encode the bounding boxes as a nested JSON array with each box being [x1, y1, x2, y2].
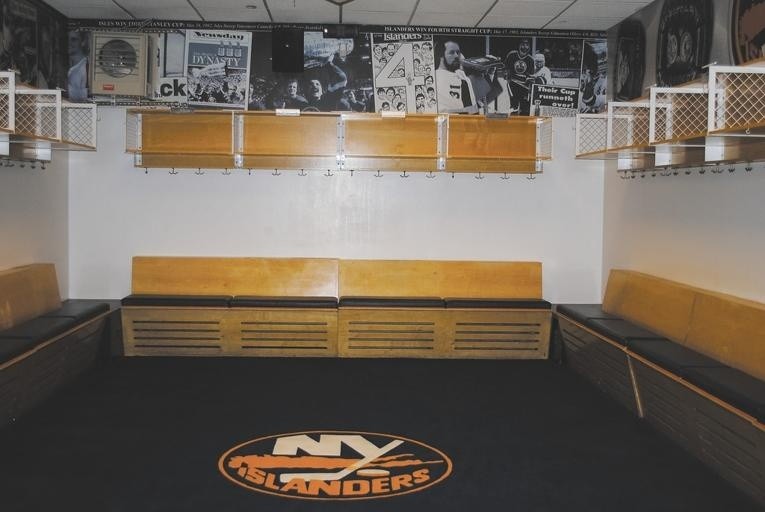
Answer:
[[322, 23, 360, 38]]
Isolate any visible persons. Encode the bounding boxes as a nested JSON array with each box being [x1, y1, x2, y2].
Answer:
[[525, 53, 551, 85], [248, 53, 375, 114], [579, 64, 600, 110], [372, 41, 435, 115], [503, 38, 534, 117], [67, 28, 90, 103], [435, 38, 479, 115]]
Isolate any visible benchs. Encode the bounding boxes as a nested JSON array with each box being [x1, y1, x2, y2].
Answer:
[[552, 269, 699, 419], [0, 263, 119, 406], [119, 255, 338, 360], [338, 260, 551, 361], [1, 337, 36, 432], [625, 289, 764, 512]]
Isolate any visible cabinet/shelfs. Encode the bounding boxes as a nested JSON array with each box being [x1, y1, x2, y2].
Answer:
[[441, 114, 551, 181], [0, 71, 98, 169], [238, 109, 339, 177], [340, 112, 442, 178], [125, 108, 236, 176], [576, 59, 765, 179]]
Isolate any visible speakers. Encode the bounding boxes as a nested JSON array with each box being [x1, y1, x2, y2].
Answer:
[[270, 32, 305, 73]]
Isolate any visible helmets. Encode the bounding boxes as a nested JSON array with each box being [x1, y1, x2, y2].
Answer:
[[534, 54, 546, 66], [519, 38, 530, 53]]
